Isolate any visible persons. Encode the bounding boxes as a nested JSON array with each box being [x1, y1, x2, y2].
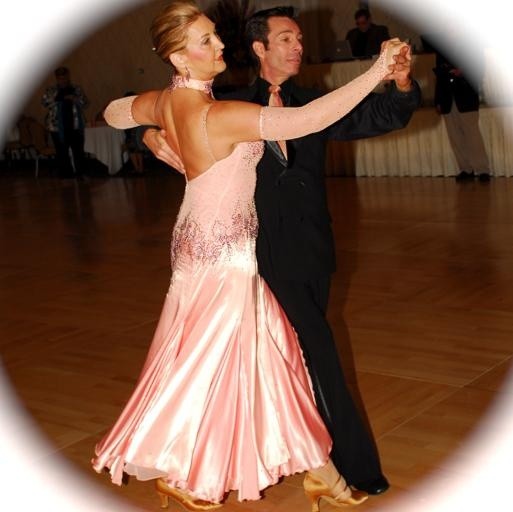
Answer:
[[131, 7, 424, 497], [84, 1, 411, 512], [344, 9, 391, 62], [121, 90, 146, 174], [38, 66, 91, 178], [426, 42, 490, 184]]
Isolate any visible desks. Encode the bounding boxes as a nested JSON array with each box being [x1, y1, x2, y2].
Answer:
[[296, 52, 512, 178], [84, 126, 131, 174]]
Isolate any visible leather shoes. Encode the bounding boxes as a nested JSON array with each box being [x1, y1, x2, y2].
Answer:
[[454, 169, 474, 181], [353, 475, 390, 495], [479, 173, 490, 182]]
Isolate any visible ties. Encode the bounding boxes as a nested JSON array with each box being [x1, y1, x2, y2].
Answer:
[[266, 84, 289, 160]]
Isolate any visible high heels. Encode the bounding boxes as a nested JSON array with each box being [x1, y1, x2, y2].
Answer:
[[156, 477, 224, 512], [303, 461, 369, 512]]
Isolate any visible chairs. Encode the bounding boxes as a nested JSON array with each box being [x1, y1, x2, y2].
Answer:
[[6, 117, 77, 178]]
